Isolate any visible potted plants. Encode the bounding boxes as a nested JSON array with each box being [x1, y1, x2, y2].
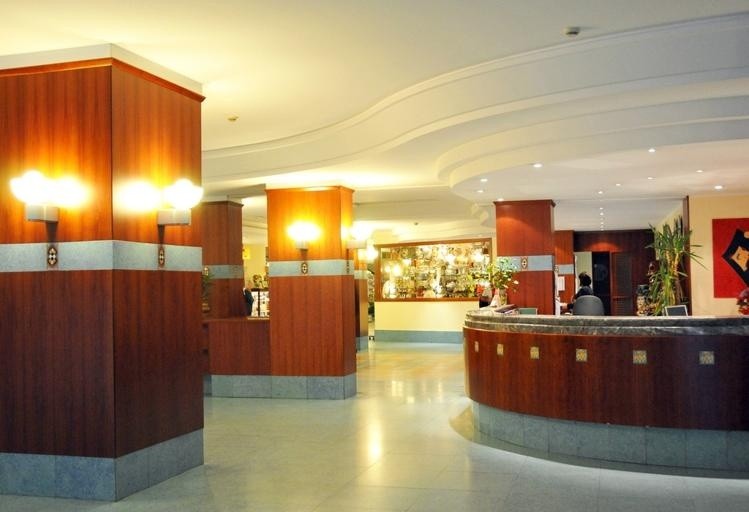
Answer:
[[373, 238, 492, 301]]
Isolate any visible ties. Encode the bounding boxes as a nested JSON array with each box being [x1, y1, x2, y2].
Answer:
[[665, 305, 688, 316]]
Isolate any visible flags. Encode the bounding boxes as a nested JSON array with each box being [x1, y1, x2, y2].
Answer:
[[498, 289, 507, 305]]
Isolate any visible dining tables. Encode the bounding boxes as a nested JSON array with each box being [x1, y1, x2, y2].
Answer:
[[201, 273, 214, 312]]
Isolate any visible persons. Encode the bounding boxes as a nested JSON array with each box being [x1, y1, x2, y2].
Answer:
[[637, 296, 646, 309], [562, 272, 593, 310], [243, 288, 254, 316]]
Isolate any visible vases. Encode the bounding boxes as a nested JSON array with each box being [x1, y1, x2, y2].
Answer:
[[486, 256, 520, 294]]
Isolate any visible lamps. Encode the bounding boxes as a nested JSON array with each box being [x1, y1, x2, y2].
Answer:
[[573, 295, 605, 316]]
